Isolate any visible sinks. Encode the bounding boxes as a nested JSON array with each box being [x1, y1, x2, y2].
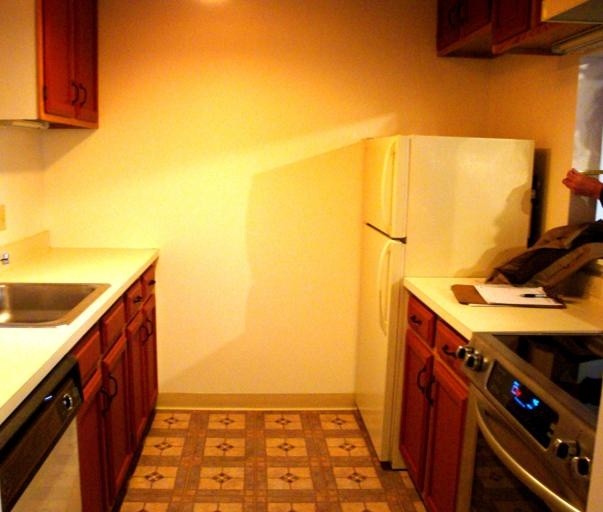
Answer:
[[3, 281, 113, 328]]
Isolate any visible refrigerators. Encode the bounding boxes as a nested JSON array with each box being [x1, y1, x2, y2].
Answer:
[[354, 135, 534, 470]]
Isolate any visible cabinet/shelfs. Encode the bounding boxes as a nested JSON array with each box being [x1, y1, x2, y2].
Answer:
[[399, 294, 468, 512], [495, 1, 602, 57], [126, 264, 159, 478], [0, 0, 100, 130], [73, 299, 134, 510], [435, 1, 494, 58]]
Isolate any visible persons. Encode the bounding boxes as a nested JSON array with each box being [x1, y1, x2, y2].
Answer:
[[562, 168, 603, 207]]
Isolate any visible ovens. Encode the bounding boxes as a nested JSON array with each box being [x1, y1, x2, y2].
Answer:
[[457, 384, 587, 512]]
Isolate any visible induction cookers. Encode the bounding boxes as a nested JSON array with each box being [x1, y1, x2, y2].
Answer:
[[455, 332, 602, 502]]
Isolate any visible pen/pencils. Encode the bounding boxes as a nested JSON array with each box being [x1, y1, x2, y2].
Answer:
[[520, 292, 553, 299]]
